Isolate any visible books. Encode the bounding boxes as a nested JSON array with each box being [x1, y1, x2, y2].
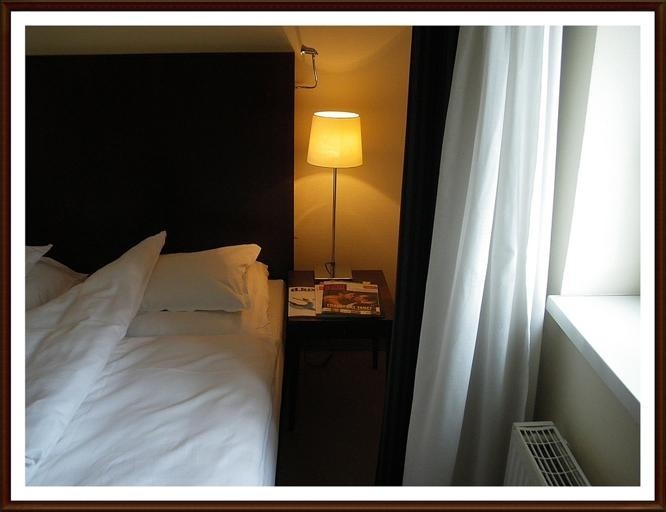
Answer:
[[287, 279, 381, 318]]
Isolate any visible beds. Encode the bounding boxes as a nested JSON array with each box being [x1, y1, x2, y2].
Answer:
[[26, 54, 293, 487]]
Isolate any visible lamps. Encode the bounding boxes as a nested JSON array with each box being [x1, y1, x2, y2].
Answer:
[[295, 110, 364, 280], [297, 44, 318, 88]]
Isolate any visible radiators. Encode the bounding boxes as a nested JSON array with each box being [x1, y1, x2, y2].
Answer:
[[504, 419, 593, 486]]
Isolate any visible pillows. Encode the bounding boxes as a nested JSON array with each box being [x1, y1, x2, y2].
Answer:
[[25, 243, 271, 337]]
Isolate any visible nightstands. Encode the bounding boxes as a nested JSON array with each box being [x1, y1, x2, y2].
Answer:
[[281, 269, 395, 431]]
